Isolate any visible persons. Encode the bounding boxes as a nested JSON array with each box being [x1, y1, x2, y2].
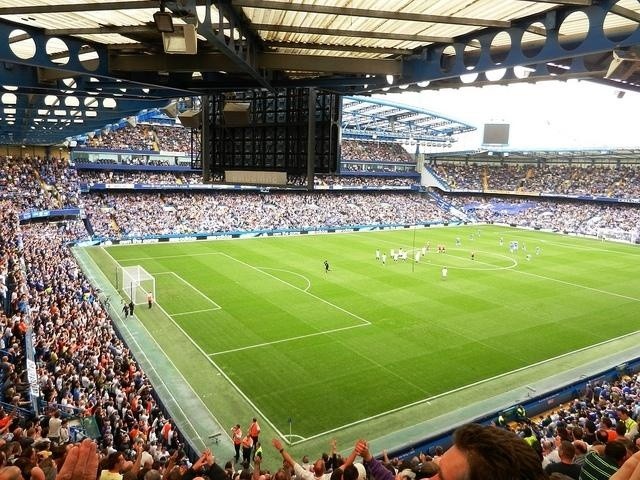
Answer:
[[214, 175, 464, 235], [427, 163, 639, 244], [341, 138, 415, 172], [321, 260, 332, 274], [440, 266, 449, 279], [368, 238, 447, 265], [455, 237, 461, 247], [85, 124, 201, 153], [0, 154, 226, 480], [509, 239, 542, 261], [469, 250, 476, 261], [231, 369, 640, 480]]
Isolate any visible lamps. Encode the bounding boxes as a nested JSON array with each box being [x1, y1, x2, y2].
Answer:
[[152, 3, 199, 55], [603, 48, 640, 81]]
